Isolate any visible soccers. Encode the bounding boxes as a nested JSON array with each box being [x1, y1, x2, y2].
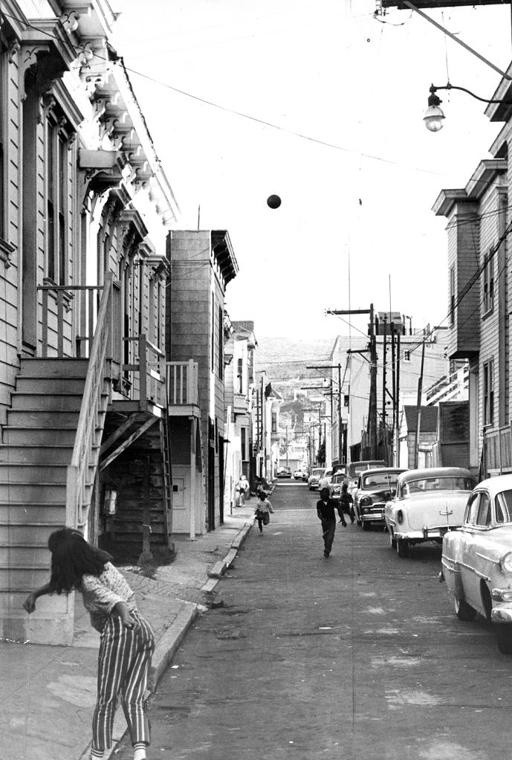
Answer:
[[267, 195, 281, 208]]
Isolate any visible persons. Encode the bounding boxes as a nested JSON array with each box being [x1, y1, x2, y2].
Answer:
[[317, 488, 347, 558], [318, 472, 328, 497], [255, 491, 275, 535], [337, 483, 355, 524], [235, 474, 250, 507], [22, 528, 155, 760]]
